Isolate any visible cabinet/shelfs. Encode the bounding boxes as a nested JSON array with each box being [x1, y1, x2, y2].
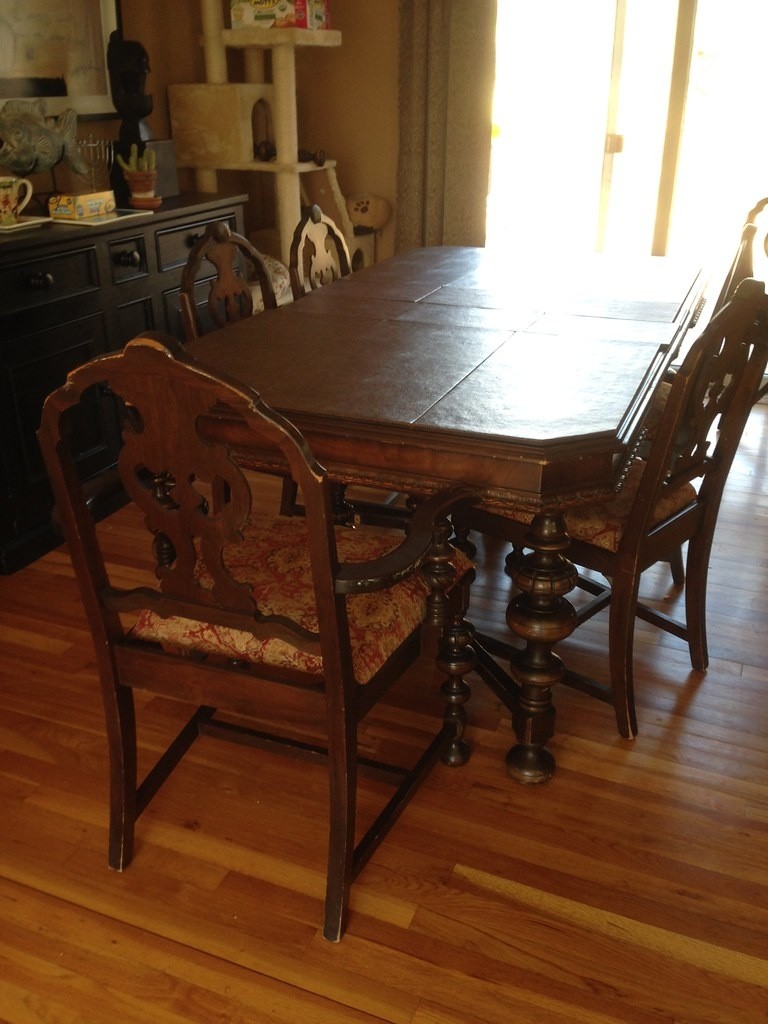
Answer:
[[168, 0, 377, 270], [0, 191, 250, 578]]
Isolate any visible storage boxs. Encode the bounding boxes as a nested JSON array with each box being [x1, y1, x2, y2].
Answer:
[[49, 190, 114, 220], [231, 0, 331, 30]]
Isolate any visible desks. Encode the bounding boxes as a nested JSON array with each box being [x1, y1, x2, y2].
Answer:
[[124, 245, 712, 783]]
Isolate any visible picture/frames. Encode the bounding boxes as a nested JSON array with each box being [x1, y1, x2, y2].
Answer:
[[0, 0, 123, 122]]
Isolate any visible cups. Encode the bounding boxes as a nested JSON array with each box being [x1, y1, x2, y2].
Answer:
[[0, 175, 33, 226]]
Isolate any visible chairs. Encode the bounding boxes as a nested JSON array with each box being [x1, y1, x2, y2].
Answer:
[[289, 205, 353, 301], [451, 278, 768, 740], [631, 222, 756, 467], [174, 222, 307, 518], [36, 331, 474, 942]]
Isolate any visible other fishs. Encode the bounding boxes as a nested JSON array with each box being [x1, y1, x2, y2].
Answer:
[[0, 98, 89, 177]]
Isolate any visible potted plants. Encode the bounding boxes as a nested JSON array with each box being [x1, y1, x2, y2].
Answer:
[[116, 144, 157, 199]]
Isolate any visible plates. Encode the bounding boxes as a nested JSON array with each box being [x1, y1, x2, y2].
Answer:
[[0, 216, 53, 233]]
